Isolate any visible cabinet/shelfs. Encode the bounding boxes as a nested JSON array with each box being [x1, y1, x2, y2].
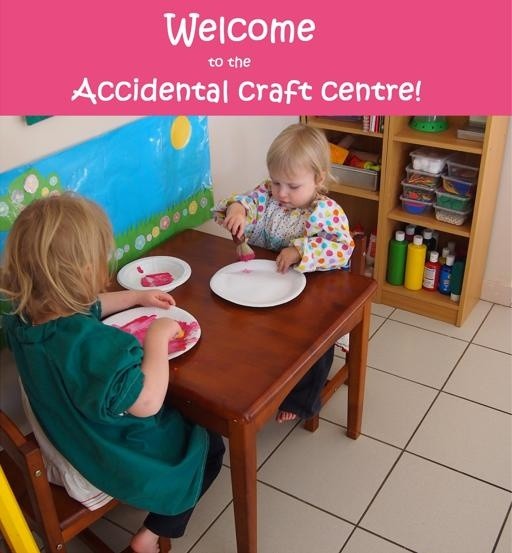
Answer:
[[299, 116, 508, 329]]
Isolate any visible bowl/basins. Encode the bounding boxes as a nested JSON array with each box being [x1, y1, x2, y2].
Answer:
[[115, 254, 193, 293]]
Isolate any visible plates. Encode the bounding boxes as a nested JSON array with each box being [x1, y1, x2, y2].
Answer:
[[91, 304, 201, 361], [209, 259, 309, 309]]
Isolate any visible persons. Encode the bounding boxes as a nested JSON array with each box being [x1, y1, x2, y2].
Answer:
[[0, 192, 226, 553], [210, 123, 355, 423]]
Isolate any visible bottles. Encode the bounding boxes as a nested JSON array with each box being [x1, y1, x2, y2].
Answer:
[[349, 221, 378, 266], [385, 223, 466, 301]]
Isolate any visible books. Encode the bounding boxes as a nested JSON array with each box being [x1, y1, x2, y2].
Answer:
[[362, 115, 384, 135]]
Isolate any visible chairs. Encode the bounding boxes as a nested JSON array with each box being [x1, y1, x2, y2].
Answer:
[[0, 409, 172, 553], [302, 234, 367, 432]]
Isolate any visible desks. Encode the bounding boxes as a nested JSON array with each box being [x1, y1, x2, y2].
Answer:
[[97, 229, 379, 553]]
